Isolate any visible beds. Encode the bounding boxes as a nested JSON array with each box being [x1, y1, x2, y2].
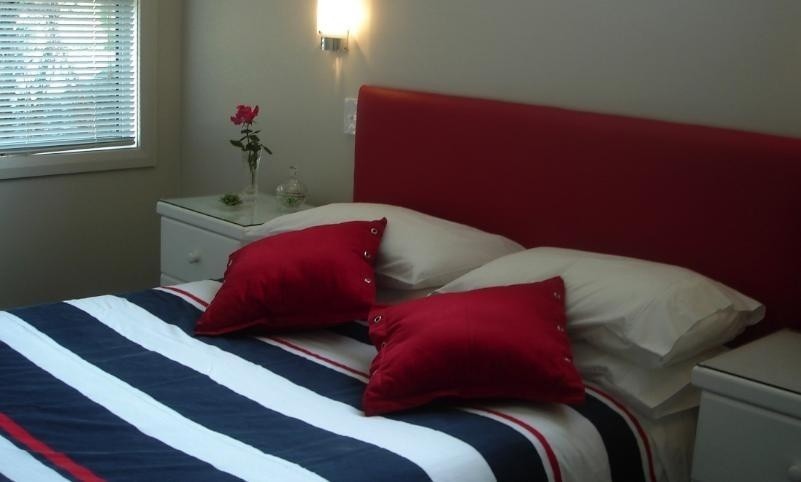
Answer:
[[1, 84, 801, 482]]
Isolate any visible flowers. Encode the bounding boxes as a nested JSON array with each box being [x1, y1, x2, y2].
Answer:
[[228, 104, 272, 184]]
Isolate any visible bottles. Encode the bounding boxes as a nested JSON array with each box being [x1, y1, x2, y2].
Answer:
[[275, 166, 305, 210]]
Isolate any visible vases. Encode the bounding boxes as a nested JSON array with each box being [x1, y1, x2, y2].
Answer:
[[239, 149, 263, 200]]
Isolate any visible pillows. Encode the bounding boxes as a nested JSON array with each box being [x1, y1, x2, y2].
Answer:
[[193, 216, 387, 335], [427, 244, 767, 365], [245, 202, 526, 293], [571, 336, 734, 419], [361, 275, 585, 416]]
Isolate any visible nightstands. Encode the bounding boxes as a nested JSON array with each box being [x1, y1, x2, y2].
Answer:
[[690, 328, 801, 481], [157, 190, 317, 285]]
